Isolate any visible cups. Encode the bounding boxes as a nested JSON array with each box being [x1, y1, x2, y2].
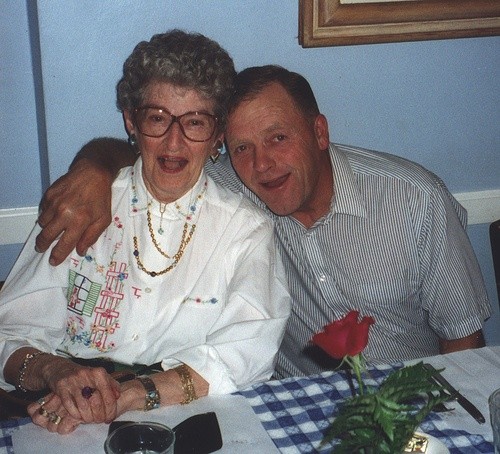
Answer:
[[489, 388, 500, 454], [104, 421, 176, 454]]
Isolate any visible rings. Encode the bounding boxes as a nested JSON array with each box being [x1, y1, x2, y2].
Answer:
[[48, 411, 63, 424], [35, 398, 45, 406], [82, 385, 97, 399], [38, 406, 49, 417]]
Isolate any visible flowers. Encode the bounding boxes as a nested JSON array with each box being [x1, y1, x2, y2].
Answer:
[[311, 309, 459, 454]]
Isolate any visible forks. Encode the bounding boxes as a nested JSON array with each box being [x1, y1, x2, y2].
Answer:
[[416, 363, 459, 401], [422, 387, 456, 412]]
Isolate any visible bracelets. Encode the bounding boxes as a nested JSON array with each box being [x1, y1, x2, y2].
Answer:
[[135, 375, 160, 412], [175, 364, 198, 405], [16, 350, 50, 392]]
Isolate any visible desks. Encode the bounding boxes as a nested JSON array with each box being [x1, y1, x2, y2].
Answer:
[[0, 347, 500, 454]]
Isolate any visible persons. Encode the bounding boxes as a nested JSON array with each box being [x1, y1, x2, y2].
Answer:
[[35, 63, 493, 381], [0, 28, 293, 430]]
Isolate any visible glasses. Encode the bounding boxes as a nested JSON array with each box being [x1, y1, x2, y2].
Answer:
[[131, 106, 221, 143]]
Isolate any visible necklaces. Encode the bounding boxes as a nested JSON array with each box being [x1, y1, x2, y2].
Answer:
[[130, 167, 208, 277]]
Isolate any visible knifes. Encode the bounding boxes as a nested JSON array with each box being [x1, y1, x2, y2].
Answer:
[[423, 363, 486, 424]]
